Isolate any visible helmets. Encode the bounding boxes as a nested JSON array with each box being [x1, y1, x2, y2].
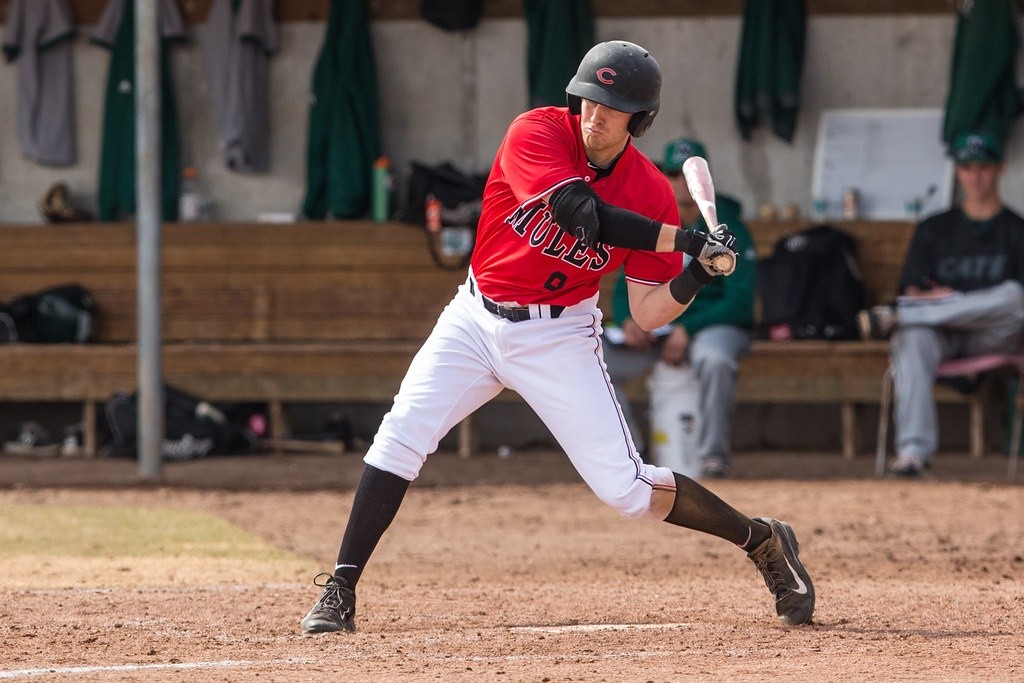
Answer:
[[565, 40, 661, 138]]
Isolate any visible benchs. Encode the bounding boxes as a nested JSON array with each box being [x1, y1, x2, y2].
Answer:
[[0, 223, 985, 457]]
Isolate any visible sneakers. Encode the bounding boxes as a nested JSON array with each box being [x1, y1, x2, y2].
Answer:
[[747, 517, 815, 624], [301, 572, 356, 634]]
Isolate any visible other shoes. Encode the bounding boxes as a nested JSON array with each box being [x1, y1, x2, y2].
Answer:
[[702, 454, 729, 478], [858, 307, 897, 338], [889, 448, 927, 474]]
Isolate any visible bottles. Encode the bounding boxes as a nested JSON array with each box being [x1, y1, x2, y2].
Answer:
[[369, 155, 393, 221], [177, 166, 203, 223], [842, 186, 859, 221]]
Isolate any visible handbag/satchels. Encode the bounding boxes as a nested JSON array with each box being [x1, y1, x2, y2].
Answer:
[[401, 162, 488, 270]]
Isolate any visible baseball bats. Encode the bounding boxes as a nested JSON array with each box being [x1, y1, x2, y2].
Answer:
[[682, 155, 734, 274]]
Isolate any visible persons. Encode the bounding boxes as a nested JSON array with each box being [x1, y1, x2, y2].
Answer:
[[856, 133, 1024, 478], [610, 139, 759, 478], [301, 40, 816, 638]]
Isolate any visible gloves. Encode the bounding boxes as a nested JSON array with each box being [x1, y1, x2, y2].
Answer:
[[685, 229, 740, 283]]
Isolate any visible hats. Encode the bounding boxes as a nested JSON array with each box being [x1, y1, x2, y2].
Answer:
[[664, 140, 705, 171], [954, 131, 1002, 164]]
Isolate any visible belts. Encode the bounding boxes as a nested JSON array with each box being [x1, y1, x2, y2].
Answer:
[[470, 279, 565, 322]]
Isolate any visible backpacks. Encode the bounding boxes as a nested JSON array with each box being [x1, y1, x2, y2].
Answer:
[[764, 226, 871, 340]]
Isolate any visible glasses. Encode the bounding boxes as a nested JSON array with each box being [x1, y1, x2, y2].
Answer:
[[668, 171, 679, 177]]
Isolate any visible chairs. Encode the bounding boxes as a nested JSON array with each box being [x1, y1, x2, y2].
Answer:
[[875, 354, 1024, 479]]
